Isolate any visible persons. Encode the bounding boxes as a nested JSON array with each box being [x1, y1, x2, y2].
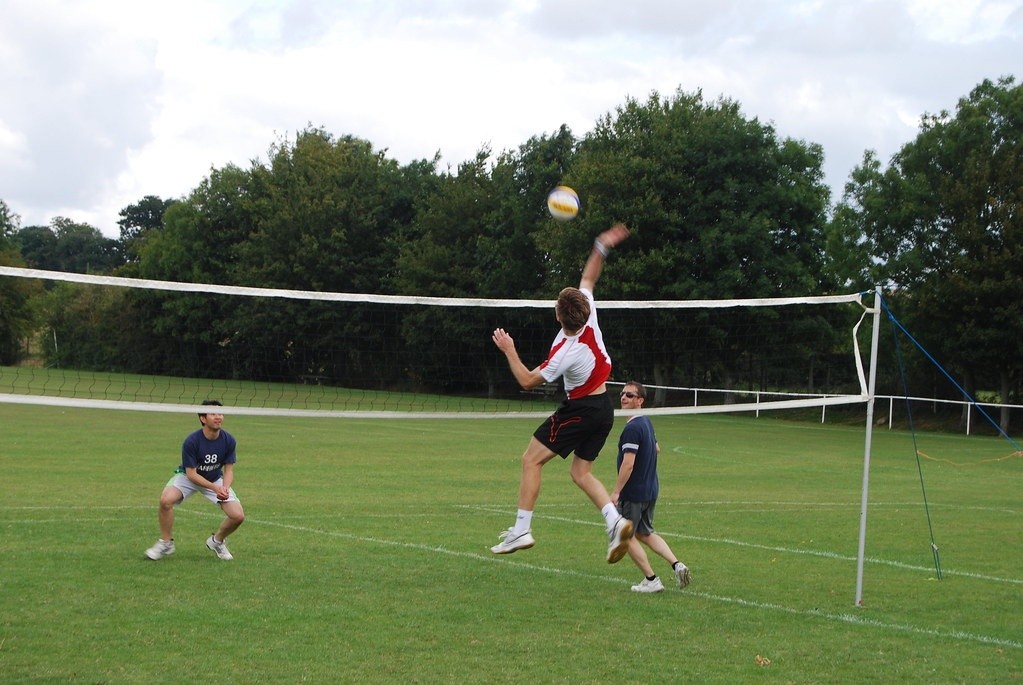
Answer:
[[610, 382, 690, 593], [145, 402, 245, 560], [491, 221, 633, 565]]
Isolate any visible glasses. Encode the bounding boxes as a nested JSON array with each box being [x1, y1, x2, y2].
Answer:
[[620, 392, 639, 399]]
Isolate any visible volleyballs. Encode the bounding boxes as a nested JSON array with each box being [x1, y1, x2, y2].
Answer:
[[548, 185, 581, 221]]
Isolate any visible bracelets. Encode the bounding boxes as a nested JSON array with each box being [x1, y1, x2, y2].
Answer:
[[593, 238, 610, 259]]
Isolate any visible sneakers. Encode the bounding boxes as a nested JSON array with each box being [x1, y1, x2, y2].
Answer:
[[489, 527, 536, 555], [630, 575, 666, 594], [205, 533, 234, 561], [674, 562, 692, 591], [144, 538, 175, 561], [605, 514, 634, 564]]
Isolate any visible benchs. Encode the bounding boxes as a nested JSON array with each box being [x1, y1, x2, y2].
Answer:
[[519, 382, 559, 400], [298, 374, 333, 386]]
